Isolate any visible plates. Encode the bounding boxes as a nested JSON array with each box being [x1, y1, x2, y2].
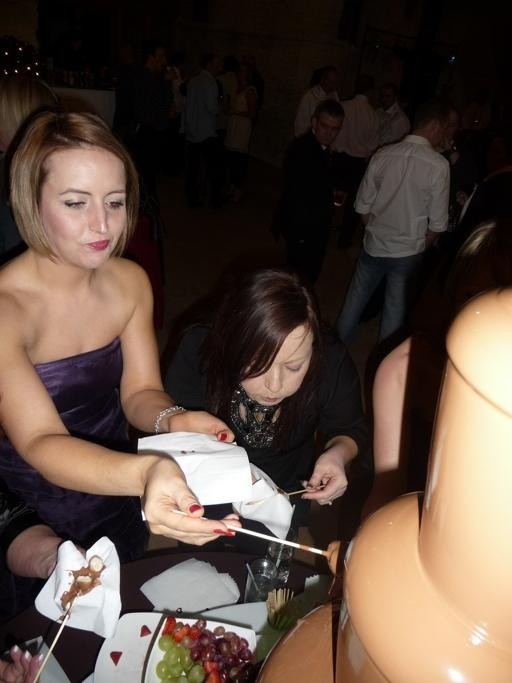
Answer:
[[144, 615, 258, 682]]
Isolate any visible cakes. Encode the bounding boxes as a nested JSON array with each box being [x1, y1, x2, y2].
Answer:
[[258, 289, 511, 683]]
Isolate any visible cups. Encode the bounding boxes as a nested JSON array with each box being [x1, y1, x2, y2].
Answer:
[[244, 558, 277, 604], [264, 528, 296, 588]]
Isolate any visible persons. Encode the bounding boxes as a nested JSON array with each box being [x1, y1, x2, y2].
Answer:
[[1, 38, 512, 627], [0, 643, 45, 683]]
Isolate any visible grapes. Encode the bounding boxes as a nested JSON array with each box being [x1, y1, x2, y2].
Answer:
[[157, 619, 251, 683]]
